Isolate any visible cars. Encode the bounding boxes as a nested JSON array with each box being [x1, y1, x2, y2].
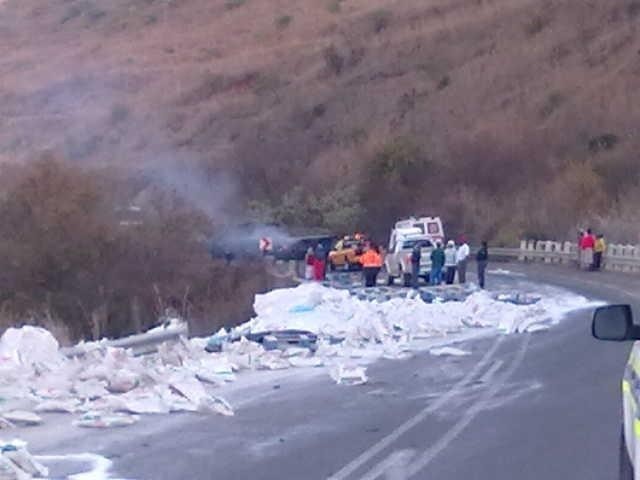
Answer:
[[591, 303, 640, 480], [208, 215, 446, 287]]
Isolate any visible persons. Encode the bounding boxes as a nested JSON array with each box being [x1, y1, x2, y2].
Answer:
[[411, 241, 421, 290], [305, 241, 328, 282], [576, 227, 607, 271], [429, 239, 470, 286], [476, 240, 488, 289], [360, 242, 402, 288]]
